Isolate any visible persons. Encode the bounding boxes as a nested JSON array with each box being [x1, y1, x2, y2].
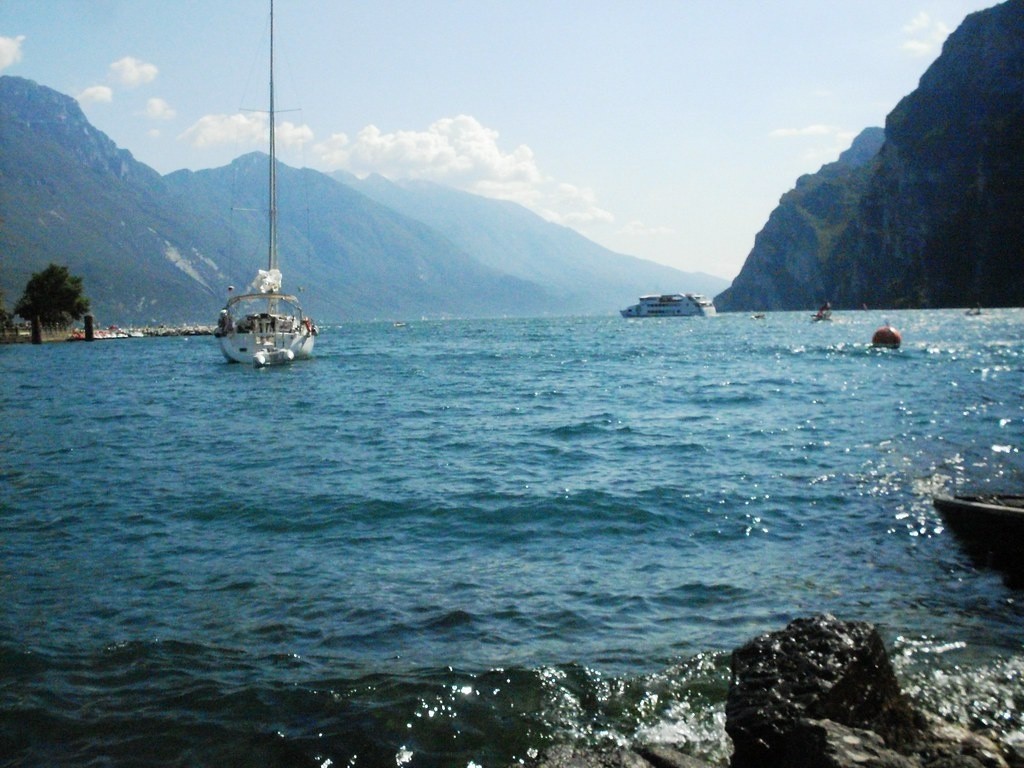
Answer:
[[218, 310, 234, 330]]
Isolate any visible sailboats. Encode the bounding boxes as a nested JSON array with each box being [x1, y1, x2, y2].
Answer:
[[216, 1, 317, 366]]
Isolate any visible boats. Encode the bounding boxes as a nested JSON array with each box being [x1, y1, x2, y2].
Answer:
[[68, 328, 143, 340], [810, 313, 831, 322], [620, 293, 717, 317], [935, 492, 1024, 570]]
